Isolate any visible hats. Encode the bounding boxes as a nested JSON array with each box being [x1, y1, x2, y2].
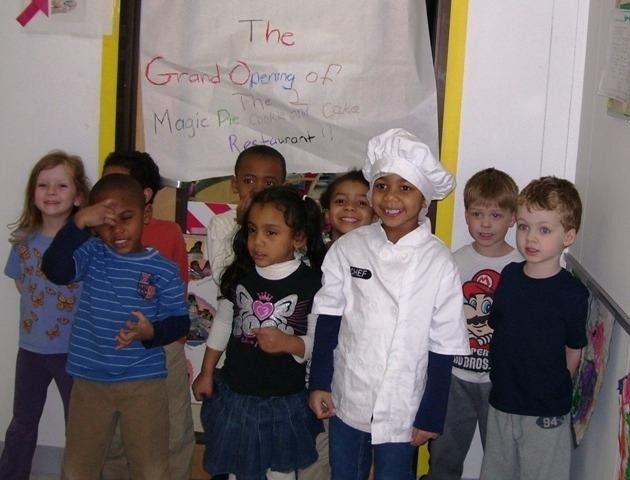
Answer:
[[361, 126, 457, 219]]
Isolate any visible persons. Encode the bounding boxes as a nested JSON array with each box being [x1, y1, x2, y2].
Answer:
[[41, 171, 191, 480], [1, 153, 88, 480], [206, 144, 312, 299], [308, 126, 471, 480], [190, 186, 326, 480], [101, 147, 196, 479], [298, 169, 377, 480], [479, 176, 591, 480], [421, 168, 526, 480]]
